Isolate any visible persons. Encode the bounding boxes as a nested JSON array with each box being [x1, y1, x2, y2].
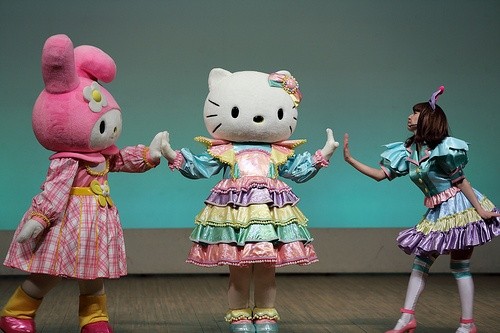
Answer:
[[343, 86, 500, 333]]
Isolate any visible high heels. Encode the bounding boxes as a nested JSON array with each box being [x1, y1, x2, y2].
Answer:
[[385, 309, 417, 333], [454, 318, 478, 333]]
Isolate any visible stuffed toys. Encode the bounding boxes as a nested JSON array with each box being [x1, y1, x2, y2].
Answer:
[[161, 68, 339, 333], [0, 35, 163, 333]]
